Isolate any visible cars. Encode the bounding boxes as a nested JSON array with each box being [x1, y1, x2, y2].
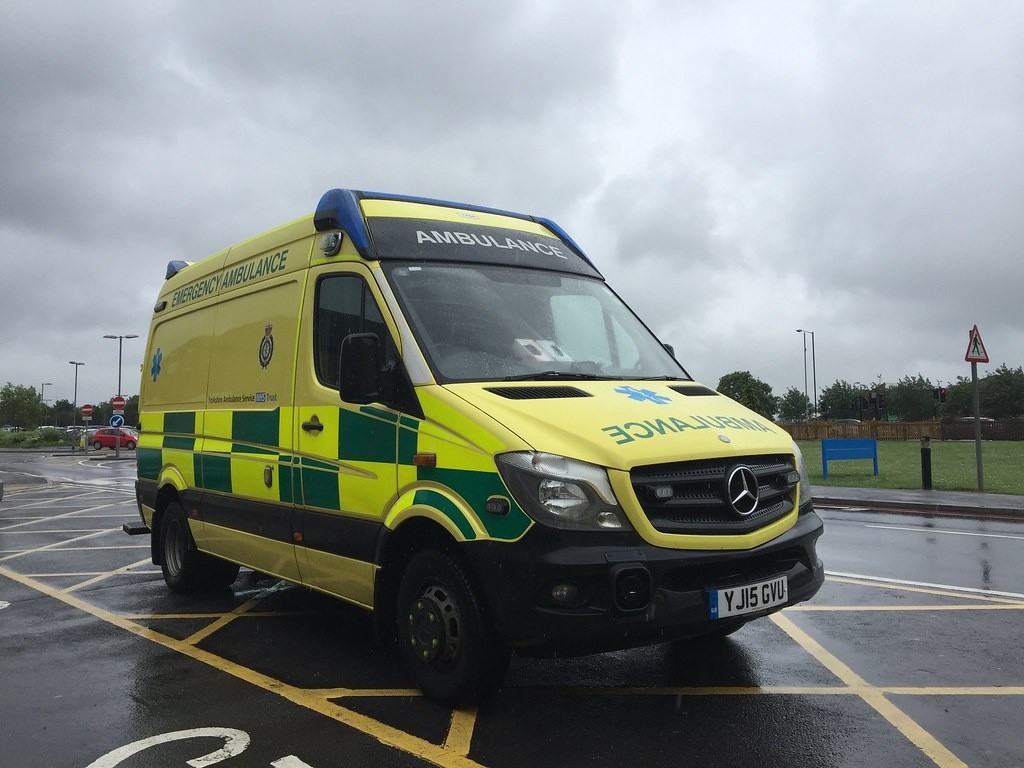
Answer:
[[37, 425, 135, 435], [2, 425, 26, 432], [92, 427, 138, 450], [835, 418, 871, 428]]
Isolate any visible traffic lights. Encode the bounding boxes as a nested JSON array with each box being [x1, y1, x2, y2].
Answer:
[[862, 395, 868, 409], [940, 387, 947, 404]]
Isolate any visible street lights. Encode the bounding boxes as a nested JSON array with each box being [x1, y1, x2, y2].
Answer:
[[40, 383, 53, 430], [68, 361, 86, 455], [795, 328, 818, 425], [102, 335, 138, 458]]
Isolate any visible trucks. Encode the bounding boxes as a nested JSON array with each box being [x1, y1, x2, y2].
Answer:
[[961, 417, 1001, 428]]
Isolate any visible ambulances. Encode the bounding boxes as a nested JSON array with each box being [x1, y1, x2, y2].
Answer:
[[122, 185, 824, 714]]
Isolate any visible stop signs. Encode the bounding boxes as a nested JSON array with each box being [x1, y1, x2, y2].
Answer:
[[113, 396, 125, 410], [82, 404, 93, 416]]
